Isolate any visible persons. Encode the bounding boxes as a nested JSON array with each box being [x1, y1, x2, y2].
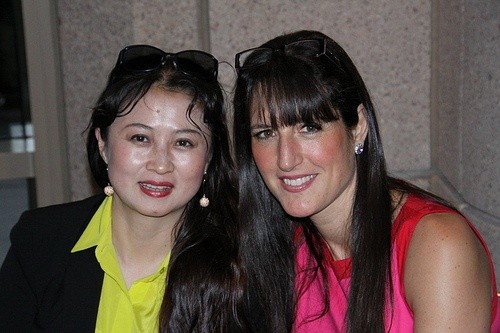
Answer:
[[232, 30, 500, 333], [1, 45, 232, 333]]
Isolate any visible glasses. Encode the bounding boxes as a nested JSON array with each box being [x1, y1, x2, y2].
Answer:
[[112, 45, 218, 85], [235, 38, 354, 79]]
